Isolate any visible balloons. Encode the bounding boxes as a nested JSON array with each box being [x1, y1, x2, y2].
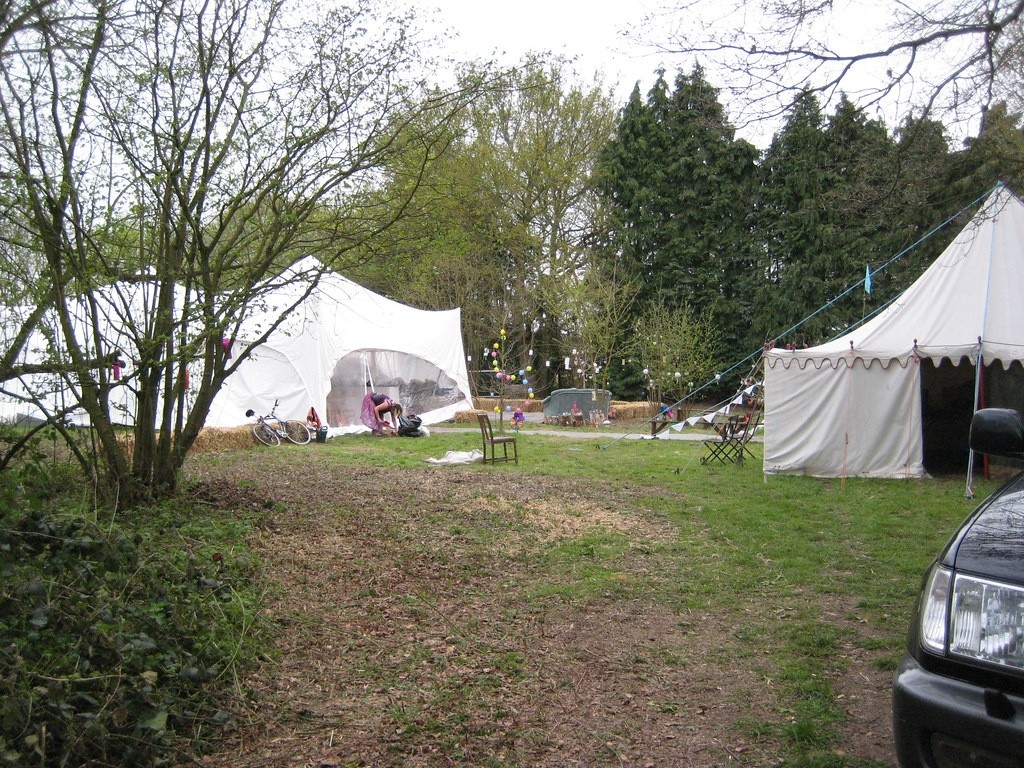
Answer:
[[493, 329, 535, 430]]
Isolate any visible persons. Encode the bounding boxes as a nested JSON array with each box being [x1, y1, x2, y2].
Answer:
[[361, 392, 402, 436]]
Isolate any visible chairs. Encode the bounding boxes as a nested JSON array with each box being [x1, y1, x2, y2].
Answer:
[[702, 411, 760, 464], [476, 415, 518, 465]]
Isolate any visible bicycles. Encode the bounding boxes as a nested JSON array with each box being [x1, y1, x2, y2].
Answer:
[[244, 398, 311, 448]]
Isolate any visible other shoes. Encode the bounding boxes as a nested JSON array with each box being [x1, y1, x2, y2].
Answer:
[[372, 430, 384, 436]]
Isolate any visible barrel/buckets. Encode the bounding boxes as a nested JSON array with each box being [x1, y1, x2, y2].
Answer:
[[316, 426, 328, 443]]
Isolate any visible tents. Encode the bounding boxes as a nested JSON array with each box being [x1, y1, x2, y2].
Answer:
[[0, 256, 475, 439], [762, 182, 1024, 498]]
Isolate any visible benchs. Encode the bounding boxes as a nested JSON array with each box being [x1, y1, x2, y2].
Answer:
[[677, 409, 711, 418], [648, 419, 764, 436]]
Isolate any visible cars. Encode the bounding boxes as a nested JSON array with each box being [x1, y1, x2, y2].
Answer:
[[892, 407, 1024, 768]]
